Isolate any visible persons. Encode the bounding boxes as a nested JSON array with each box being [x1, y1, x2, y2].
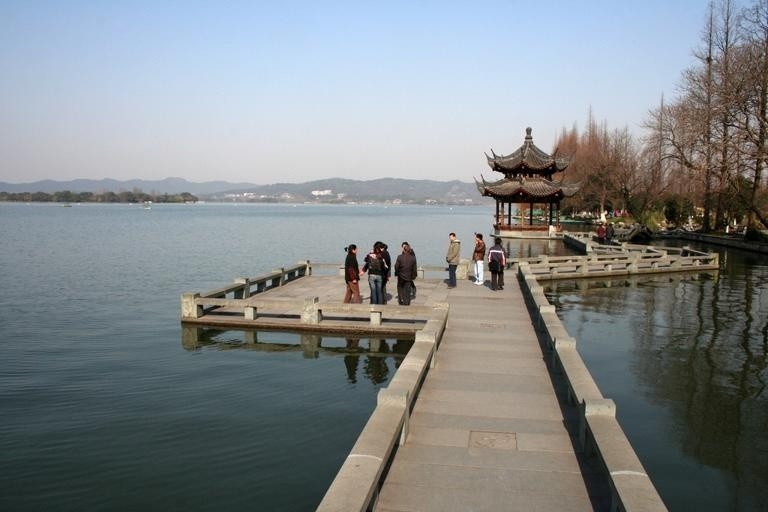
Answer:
[[445, 233, 462, 288], [342, 244, 361, 303], [374, 241, 392, 304], [393, 246, 416, 305], [471, 232, 485, 286], [400, 242, 417, 297], [360, 247, 387, 304], [606, 222, 614, 246], [486, 237, 507, 291], [597, 222, 606, 243]]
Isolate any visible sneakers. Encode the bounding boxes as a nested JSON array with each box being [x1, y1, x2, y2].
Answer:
[[447, 281, 456, 288], [492, 285, 504, 291], [473, 280, 483, 286]]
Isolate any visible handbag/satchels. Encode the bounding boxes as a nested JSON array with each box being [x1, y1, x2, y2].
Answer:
[[488, 260, 499, 271]]
